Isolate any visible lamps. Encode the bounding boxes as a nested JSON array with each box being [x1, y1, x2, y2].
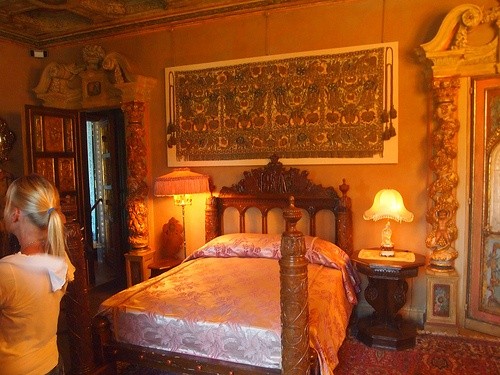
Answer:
[[363, 189, 414, 257], [153, 168, 212, 259]]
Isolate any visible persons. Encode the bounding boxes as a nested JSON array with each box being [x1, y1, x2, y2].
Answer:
[[0, 174, 76, 375]]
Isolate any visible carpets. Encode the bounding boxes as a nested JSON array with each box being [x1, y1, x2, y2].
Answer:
[[334, 331, 500, 375]]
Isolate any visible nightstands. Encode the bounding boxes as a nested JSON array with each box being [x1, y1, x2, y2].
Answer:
[[147, 260, 181, 279], [351, 248, 427, 350]]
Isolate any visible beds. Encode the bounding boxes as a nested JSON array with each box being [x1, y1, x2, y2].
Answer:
[[93, 154, 354, 375]]
